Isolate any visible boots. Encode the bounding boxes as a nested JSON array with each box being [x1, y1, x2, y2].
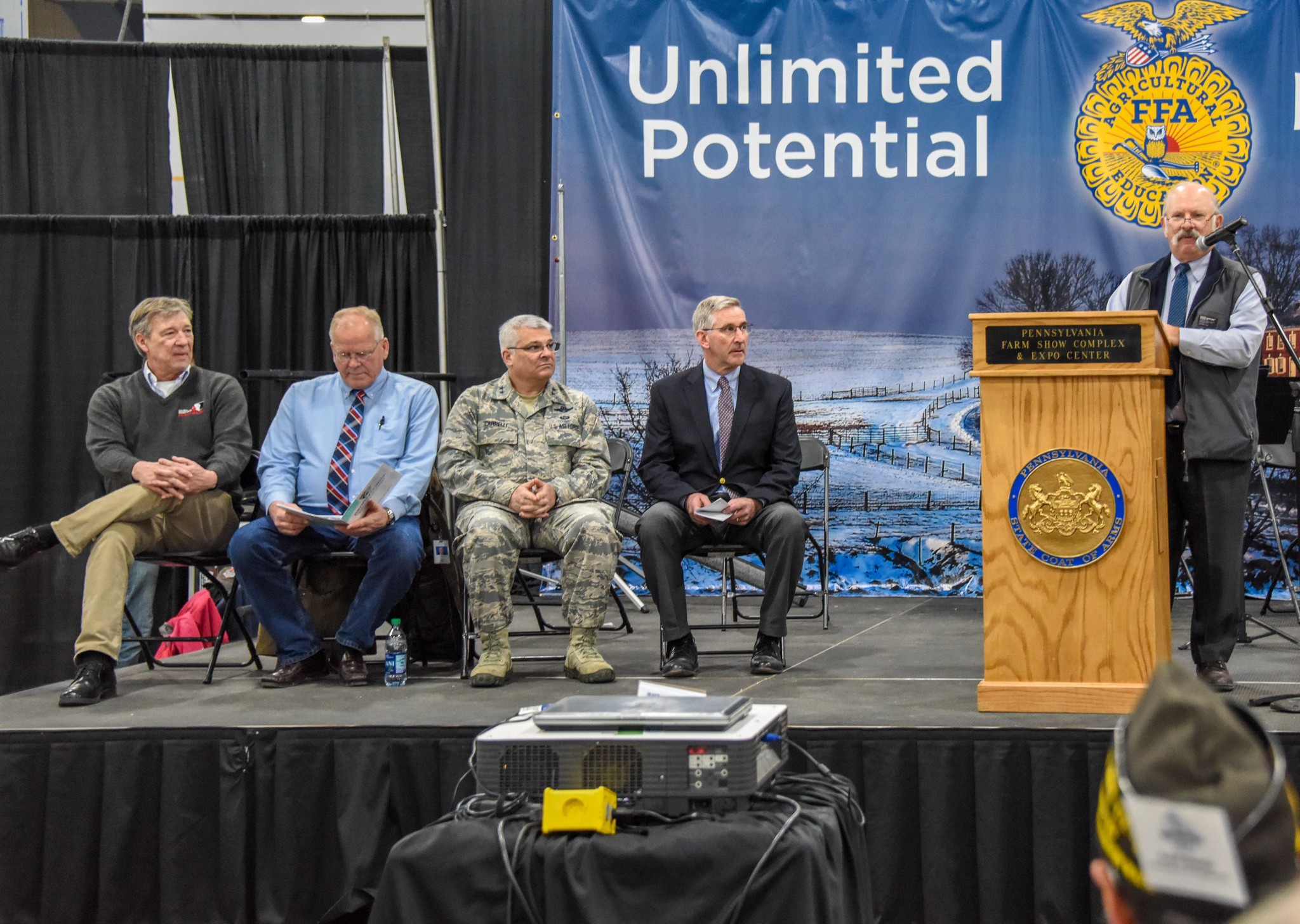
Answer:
[[564, 625, 617, 684], [470, 626, 512, 685]]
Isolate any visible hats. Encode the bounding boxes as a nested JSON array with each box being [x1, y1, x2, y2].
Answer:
[[1097, 657, 1297, 924]]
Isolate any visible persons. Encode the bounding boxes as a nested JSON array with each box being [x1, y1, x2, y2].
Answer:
[[227, 306, 440, 688], [635, 295, 808, 677], [1086, 662, 1300, 924], [1105, 181, 1269, 692], [0, 296, 253, 706], [435, 313, 622, 687]]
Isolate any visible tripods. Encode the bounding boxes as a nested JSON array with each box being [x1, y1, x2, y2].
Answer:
[[1179, 615, 1300, 650]]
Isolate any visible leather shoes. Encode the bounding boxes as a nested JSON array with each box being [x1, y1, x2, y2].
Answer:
[[1193, 656, 1233, 690], [60, 660, 117, 706], [0, 524, 43, 567], [750, 633, 782, 673], [263, 654, 329, 688], [330, 643, 368, 685], [661, 633, 700, 675]]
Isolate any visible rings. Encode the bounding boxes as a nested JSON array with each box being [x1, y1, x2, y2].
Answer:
[[736, 518, 738, 522]]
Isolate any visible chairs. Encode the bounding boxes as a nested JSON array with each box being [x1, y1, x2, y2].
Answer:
[[287, 481, 432, 674], [114, 448, 266, 686], [654, 535, 789, 674], [459, 435, 635, 683], [716, 433, 836, 633]]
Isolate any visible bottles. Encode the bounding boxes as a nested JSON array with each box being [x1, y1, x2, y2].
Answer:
[[384, 618, 407, 687]]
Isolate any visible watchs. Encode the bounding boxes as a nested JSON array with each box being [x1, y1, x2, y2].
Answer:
[[384, 507, 395, 526]]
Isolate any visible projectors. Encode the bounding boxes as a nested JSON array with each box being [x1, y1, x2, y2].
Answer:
[[476, 706, 789, 815]]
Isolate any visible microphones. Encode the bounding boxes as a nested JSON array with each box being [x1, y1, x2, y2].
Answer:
[[1196, 217, 1248, 251]]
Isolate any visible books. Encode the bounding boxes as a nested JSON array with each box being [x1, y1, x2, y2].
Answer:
[[276, 461, 403, 526]]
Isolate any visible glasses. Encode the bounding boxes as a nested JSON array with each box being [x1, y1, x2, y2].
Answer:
[[508, 342, 562, 353], [1163, 209, 1219, 231], [333, 339, 383, 363], [703, 323, 753, 336]]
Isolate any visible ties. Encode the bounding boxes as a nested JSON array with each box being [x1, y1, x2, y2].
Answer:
[[328, 389, 366, 515], [1163, 261, 1188, 409], [718, 377, 743, 500]]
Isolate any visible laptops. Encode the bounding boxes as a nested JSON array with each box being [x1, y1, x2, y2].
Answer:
[[534, 697, 751, 731]]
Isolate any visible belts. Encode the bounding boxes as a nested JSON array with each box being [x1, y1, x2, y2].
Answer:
[[1165, 424, 1184, 436]]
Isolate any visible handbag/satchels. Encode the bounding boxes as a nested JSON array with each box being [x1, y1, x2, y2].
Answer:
[[153, 590, 228, 659]]
